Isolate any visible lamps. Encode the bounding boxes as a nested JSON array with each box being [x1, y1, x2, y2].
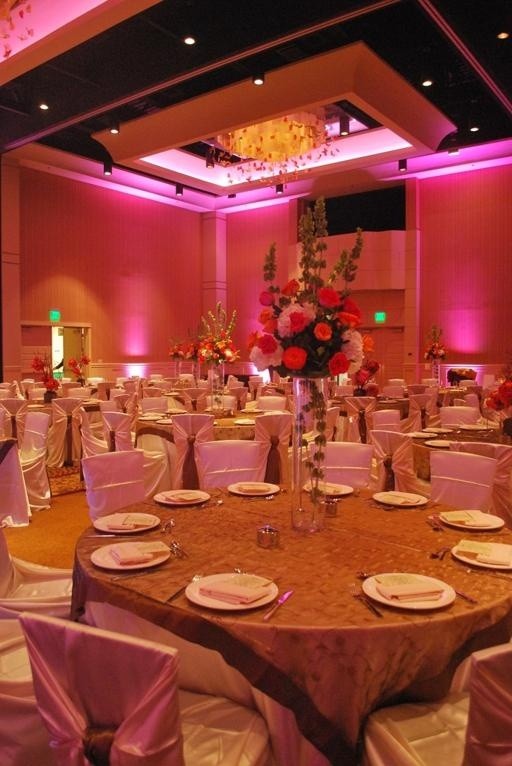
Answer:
[[398, 159, 407, 172], [206, 107, 339, 188], [275, 183, 283, 195], [448, 140, 459, 156], [104, 164, 112, 176], [252, 75, 264, 85], [110, 123, 120, 134], [340, 117, 350, 136], [176, 183, 183, 197]]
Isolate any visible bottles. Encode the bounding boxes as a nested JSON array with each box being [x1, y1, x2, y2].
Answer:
[[257, 526, 279, 549], [320, 500, 337, 517]]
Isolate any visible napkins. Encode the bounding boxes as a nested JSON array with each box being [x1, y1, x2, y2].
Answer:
[[199, 579, 268, 604], [376, 579, 442, 602], [453, 539, 512, 567], [311, 479, 342, 494], [237, 483, 268, 495], [381, 489, 419, 505], [442, 510, 489, 528], [165, 489, 202, 502], [110, 541, 150, 566], [107, 512, 155, 529]]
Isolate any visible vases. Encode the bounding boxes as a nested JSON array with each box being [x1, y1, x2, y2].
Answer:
[[174, 361, 182, 377], [213, 362, 224, 384], [432, 358, 441, 386], [192, 359, 201, 380]]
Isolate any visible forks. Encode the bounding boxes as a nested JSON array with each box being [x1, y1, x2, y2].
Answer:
[[348, 582, 383, 619]]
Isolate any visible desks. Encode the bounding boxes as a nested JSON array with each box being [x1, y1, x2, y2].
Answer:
[[2, 438, 33, 525], [78, 486, 512, 734]]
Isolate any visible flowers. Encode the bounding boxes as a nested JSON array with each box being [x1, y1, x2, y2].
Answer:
[[31, 356, 60, 391], [246, 196, 373, 378], [196, 300, 241, 364], [424, 325, 448, 360], [68, 356, 91, 374], [169, 337, 186, 362], [187, 323, 206, 361]]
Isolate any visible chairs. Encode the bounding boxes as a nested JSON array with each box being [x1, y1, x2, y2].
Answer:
[[16, 613, 267, 766], [430, 452, 499, 511], [81, 448, 148, 522], [192, 441, 265, 488], [1, 371, 511, 465], [1, 616, 54, 766], [20, 412, 50, 509], [1, 527, 76, 613], [361, 638, 512, 765], [321, 442, 378, 488]]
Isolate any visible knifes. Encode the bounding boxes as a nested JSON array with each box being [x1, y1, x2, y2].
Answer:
[[263, 590, 295, 621]]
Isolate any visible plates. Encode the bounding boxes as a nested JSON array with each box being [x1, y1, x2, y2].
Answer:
[[363, 572, 457, 610], [440, 512, 512, 569], [184, 572, 278, 611], [233, 420, 255, 424], [152, 481, 428, 507], [138, 413, 172, 425], [405, 426, 493, 448], [241, 409, 262, 413], [90, 513, 170, 570]]
